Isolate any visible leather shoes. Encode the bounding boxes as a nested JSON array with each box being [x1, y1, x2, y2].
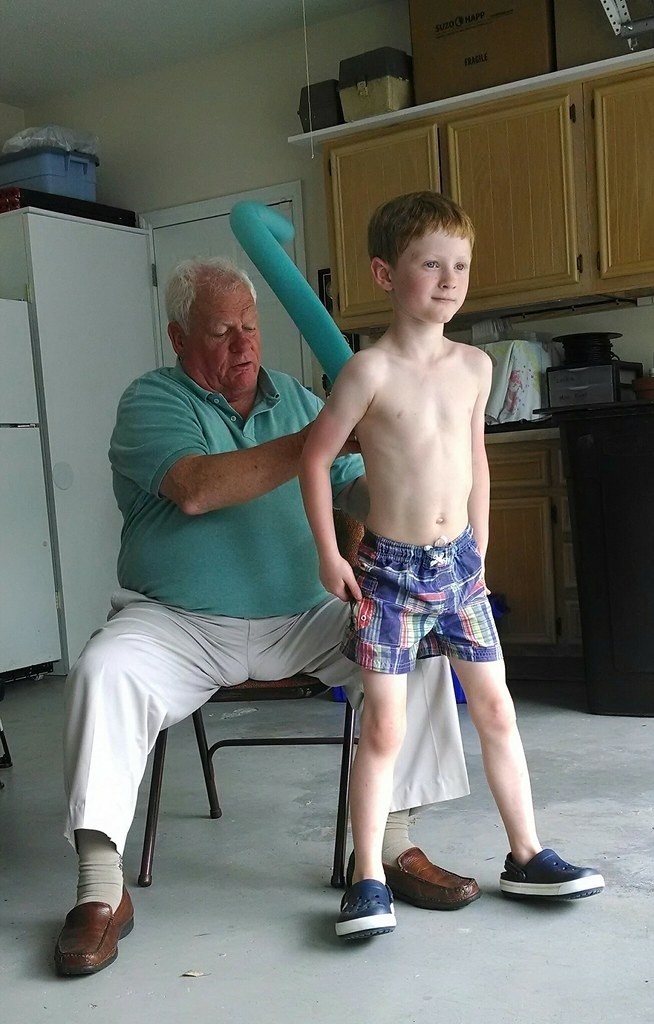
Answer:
[[54, 887, 135, 980], [346, 847, 481, 910]]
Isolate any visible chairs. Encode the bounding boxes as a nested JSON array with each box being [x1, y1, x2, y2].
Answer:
[[136, 509, 366, 891]]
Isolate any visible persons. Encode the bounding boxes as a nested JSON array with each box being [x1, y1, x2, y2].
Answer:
[[299, 190, 607, 942], [52, 254, 481, 977]]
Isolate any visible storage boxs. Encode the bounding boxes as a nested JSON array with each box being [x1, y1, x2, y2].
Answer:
[[298, 79, 346, 134], [336, 594, 511, 704], [0, 145, 101, 204], [337, 46, 414, 122], [0, 187, 136, 228], [554, 0, 654, 67], [408, 0, 557, 107]]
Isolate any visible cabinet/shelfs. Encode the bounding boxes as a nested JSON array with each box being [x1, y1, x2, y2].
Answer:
[[483, 425, 588, 682], [318, 80, 598, 336], [583, 62, 654, 298]]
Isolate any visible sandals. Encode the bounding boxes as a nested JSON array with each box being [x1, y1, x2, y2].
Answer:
[[500, 849, 605, 900], [335, 879, 396, 944]]
